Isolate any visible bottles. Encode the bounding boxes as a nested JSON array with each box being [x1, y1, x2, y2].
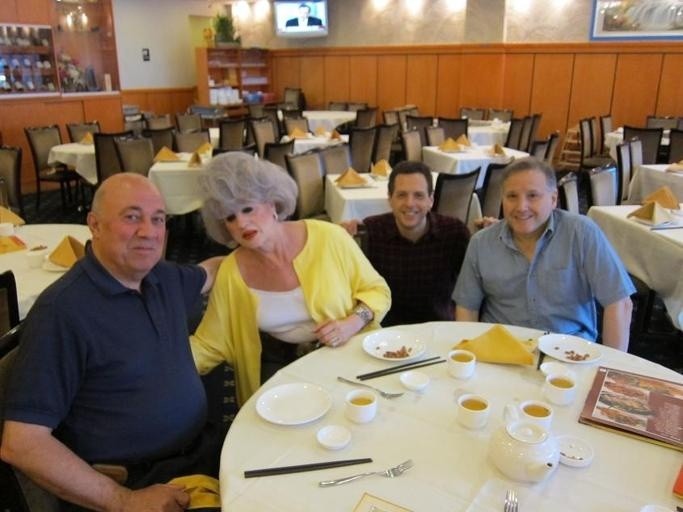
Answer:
[[0, 26, 58, 94]]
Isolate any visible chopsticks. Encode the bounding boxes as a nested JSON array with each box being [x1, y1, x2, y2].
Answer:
[[537, 330, 550, 371], [243, 458, 373, 479], [356, 357, 446, 382]]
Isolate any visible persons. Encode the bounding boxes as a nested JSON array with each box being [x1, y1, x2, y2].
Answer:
[[286, 3, 322, 27], [0, 172, 226, 512], [185, 149, 394, 411], [341, 161, 501, 327], [451, 157, 638, 352]]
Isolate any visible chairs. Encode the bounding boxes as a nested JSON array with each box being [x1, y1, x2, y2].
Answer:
[[3, 89, 681, 225], [0, 320, 71, 511]]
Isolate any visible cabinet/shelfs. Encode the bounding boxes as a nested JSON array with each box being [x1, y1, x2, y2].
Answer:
[[0, 21, 61, 92], [195, 45, 273, 106]]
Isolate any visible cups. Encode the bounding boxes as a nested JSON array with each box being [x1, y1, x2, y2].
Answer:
[[345, 389, 378, 423], [543, 373, 577, 406], [456, 393, 490, 429], [520, 400, 554, 431], [0, 223, 15, 237], [447, 350, 477, 378]]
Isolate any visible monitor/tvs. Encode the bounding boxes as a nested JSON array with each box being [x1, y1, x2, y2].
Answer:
[[271, 1, 328, 39]]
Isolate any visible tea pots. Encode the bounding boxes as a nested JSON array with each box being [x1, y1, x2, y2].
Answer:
[[488, 403, 560, 482]]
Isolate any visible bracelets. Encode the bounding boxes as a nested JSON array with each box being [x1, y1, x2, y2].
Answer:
[[354, 305, 371, 324]]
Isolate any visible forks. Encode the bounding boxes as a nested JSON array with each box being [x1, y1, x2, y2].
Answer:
[[337, 376, 404, 400], [319, 458, 414, 488], [502, 488, 518, 512]]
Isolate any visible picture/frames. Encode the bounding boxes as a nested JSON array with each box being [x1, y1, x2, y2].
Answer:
[[588, 0, 683, 41]]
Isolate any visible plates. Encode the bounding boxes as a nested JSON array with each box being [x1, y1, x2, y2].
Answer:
[[42, 261, 68, 272], [256, 381, 350, 450], [399, 370, 428, 392], [361, 330, 428, 362], [536, 334, 604, 365]]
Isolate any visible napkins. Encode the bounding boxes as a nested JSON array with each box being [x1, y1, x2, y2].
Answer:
[[51, 235, 86, 266], [453, 324, 536, 366], [1, 234, 26, 255]]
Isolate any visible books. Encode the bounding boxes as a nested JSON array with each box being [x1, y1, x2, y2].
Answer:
[[577, 366, 683, 451]]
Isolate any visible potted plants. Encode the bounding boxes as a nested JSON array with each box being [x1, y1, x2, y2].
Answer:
[[210, 13, 241, 47]]
